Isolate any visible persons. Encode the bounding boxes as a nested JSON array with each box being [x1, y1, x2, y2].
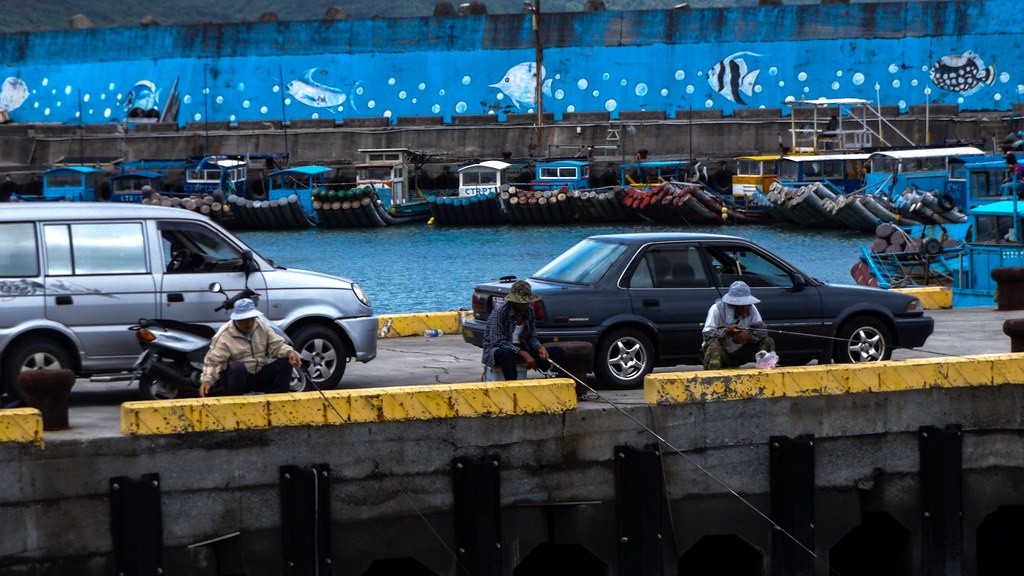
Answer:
[[482, 280, 598, 401], [700, 280, 774, 370], [200, 299, 302, 396], [1000, 153, 1024, 196]]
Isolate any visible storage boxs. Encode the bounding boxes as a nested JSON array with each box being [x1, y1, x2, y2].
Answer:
[[483, 362, 527, 382]]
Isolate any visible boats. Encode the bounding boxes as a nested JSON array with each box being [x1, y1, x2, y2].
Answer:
[[8, 97, 1024, 230], [852, 165, 1024, 309]]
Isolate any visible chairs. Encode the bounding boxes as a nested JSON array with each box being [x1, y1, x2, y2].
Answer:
[[666, 265, 695, 286]]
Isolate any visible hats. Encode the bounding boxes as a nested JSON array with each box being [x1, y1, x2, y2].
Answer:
[[504, 280, 542, 302], [722, 281, 761, 304], [229, 298, 264, 320], [1003, 154, 1015, 160]]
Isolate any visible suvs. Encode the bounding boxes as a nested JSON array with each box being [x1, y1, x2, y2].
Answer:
[[0, 201, 380, 407]]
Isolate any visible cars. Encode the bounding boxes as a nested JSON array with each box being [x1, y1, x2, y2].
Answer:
[[461, 233, 936, 392]]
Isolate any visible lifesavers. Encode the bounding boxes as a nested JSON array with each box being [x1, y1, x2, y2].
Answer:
[[409, 173, 432, 196], [600, 171, 618, 189], [332, 176, 345, 191], [98, 181, 111, 202], [712, 169, 733, 194], [516, 171, 534, 190], [938, 194, 954, 211], [248, 177, 268, 200], [432, 173, 459, 196], [0, 181, 23, 202], [166, 182, 184, 197]]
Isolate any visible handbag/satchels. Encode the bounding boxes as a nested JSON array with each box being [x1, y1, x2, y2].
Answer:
[[755, 350, 779, 369]]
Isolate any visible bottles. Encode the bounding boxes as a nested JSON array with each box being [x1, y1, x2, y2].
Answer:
[[424, 329, 445, 337]]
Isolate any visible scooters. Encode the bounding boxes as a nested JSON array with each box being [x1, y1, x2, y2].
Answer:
[[125, 260, 312, 401]]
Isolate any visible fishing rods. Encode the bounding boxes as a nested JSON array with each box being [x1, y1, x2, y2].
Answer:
[[699, 322, 960, 358], [545, 355, 841, 576], [289, 357, 471, 576]]
